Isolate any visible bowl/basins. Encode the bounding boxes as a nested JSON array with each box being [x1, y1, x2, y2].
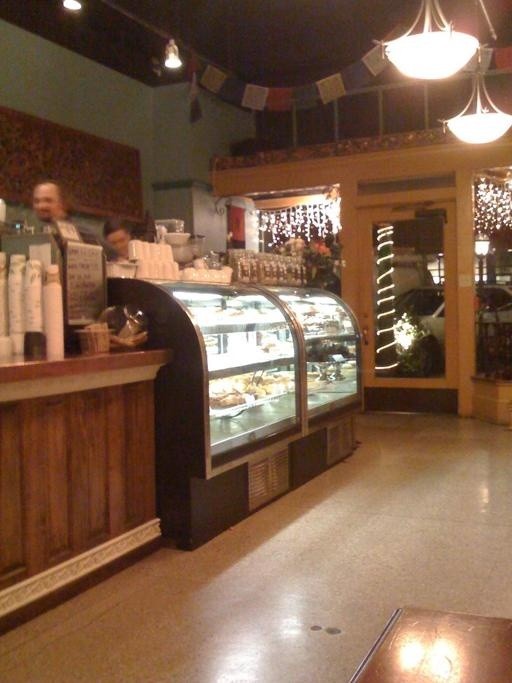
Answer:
[[165, 232, 190, 245], [180, 264, 234, 284]]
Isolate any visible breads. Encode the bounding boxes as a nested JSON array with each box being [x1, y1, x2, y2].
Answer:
[[209, 372, 291, 408]]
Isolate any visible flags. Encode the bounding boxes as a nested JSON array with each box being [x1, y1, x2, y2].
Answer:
[[188, 53, 202, 123], [362, 47, 391, 78], [340, 60, 369, 91], [385, 25, 410, 42], [315, 73, 345, 105], [295, 84, 319, 110], [465, 49, 494, 73], [200, 65, 293, 111], [494, 48, 511, 70]]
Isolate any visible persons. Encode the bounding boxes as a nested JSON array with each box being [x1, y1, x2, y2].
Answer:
[[101, 218, 141, 278], [31, 180, 120, 265]]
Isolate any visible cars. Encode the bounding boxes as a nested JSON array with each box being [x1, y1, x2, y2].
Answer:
[[393, 284, 512, 354]]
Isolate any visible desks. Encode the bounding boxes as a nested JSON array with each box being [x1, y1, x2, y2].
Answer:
[[351, 603, 512, 682]]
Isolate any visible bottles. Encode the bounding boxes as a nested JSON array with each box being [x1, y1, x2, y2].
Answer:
[[226, 247, 308, 287]]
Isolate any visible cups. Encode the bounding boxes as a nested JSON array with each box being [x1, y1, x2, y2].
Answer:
[[41, 262, 66, 360], [1, 250, 11, 366], [24, 257, 43, 333], [7, 251, 27, 366], [128, 238, 180, 279]]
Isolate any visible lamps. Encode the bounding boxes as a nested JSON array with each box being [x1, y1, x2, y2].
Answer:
[[382, 1, 485, 81], [444, 49, 512, 146], [158, 36, 186, 71]]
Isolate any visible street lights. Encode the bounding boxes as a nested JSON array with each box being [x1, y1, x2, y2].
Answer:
[[474, 229, 491, 376]]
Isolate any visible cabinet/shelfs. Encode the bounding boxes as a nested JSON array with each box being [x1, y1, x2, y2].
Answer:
[[104, 277, 365, 480]]
